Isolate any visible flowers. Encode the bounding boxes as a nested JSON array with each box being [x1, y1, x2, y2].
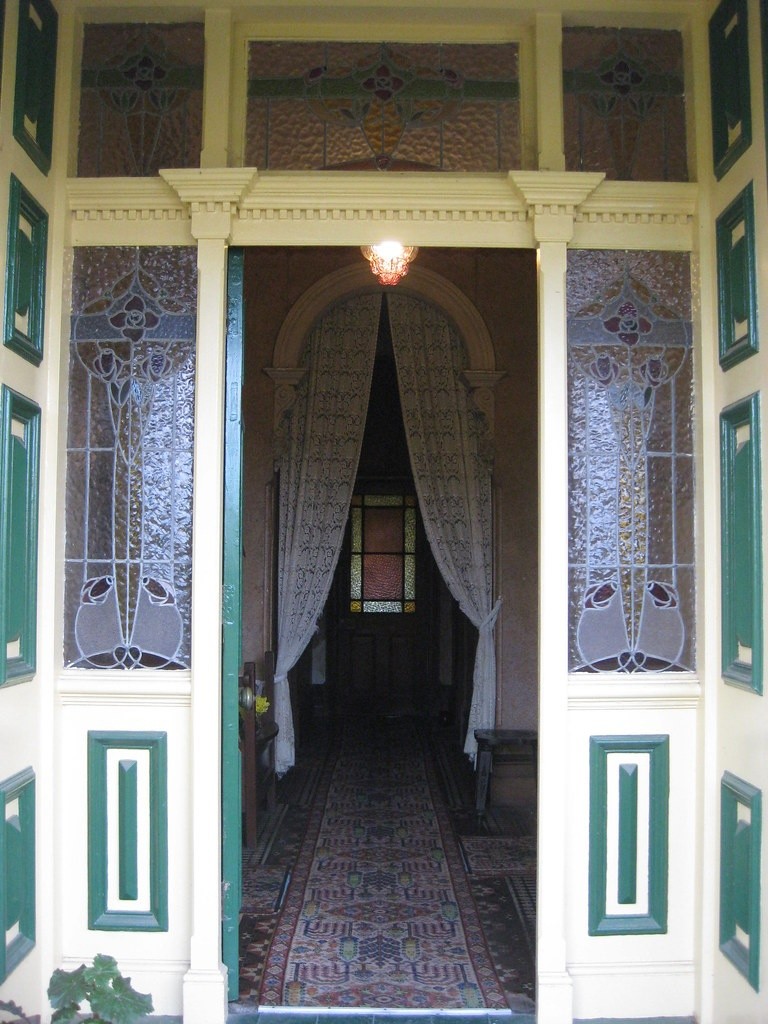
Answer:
[[255, 694, 270, 719]]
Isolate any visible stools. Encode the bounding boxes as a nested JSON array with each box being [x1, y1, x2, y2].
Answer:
[[473, 728, 538, 838]]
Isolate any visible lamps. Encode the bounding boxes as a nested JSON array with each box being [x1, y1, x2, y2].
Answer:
[[361, 246, 418, 287]]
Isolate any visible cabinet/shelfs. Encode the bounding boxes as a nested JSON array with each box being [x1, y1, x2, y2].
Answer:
[[239, 651, 280, 850]]
